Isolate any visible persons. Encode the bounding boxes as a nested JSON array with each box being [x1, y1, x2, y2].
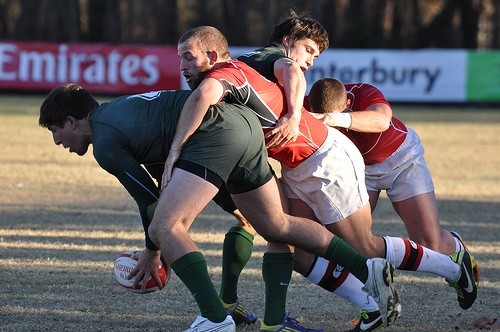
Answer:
[[218, 14, 329, 332], [159, 26, 477, 332], [39, 84, 402, 332], [301, 77, 481, 284]]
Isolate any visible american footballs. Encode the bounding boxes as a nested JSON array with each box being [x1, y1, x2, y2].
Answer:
[[112, 249, 167, 294]]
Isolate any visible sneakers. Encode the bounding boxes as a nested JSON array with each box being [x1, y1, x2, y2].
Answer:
[[449, 230, 480, 282], [445, 251, 478, 310], [362, 258, 402, 327], [345, 310, 383, 332], [183, 315, 236, 332], [259, 316, 323, 332], [218, 295, 257, 324]]
